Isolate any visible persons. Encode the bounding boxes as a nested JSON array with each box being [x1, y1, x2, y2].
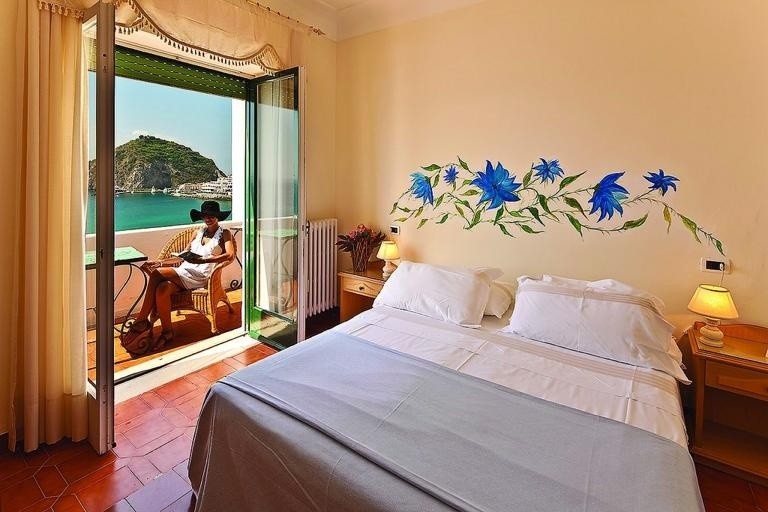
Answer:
[[133, 201, 234, 352]]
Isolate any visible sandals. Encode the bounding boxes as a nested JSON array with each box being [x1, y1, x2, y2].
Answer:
[[121, 319, 151, 346], [153, 328, 175, 351]]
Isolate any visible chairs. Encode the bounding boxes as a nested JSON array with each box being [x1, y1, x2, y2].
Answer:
[[149, 225, 238, 335]]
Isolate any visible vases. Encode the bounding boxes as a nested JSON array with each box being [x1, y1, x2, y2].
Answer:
[[349, 244, 374, 272]]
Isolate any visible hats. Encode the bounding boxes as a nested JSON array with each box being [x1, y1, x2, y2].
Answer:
[[190, 201, 231, 222]]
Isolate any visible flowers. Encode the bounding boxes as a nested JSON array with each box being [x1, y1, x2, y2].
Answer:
[[335, 223, 388, 272], [385, 152, 732, 258]]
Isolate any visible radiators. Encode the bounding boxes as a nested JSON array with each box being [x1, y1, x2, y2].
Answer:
[[304, 218, 337, 319]]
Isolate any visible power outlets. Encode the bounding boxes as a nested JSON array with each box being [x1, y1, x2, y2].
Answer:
[[700, 257, 731, 275], [388, 225, 400, 236]]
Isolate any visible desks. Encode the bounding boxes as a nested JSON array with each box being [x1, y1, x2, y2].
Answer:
[[85, 245, 151, 356], [259, 230, 297, 310]]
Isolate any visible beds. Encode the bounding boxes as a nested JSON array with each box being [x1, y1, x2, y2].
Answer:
[[184, 302, 699, 511]]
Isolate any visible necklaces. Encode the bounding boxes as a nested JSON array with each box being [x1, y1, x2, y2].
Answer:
[[205, 231, 214, 238]]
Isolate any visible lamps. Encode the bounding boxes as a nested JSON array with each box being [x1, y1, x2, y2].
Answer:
[[687, 283, 739, 348], [376, 241, 400, 278]]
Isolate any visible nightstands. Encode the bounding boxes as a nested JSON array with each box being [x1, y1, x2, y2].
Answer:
[[686, 321, 768, 486], [336, 263, 386, 324]]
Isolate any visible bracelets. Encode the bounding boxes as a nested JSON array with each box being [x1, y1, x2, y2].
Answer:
[[160, 261, 163, 267]]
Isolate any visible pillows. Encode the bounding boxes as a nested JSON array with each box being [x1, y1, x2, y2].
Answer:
[[371, 260, 504, 330], [483, 282, 515, 320], [495, 274, 693, 385], [540, 272, 666, 311]]
